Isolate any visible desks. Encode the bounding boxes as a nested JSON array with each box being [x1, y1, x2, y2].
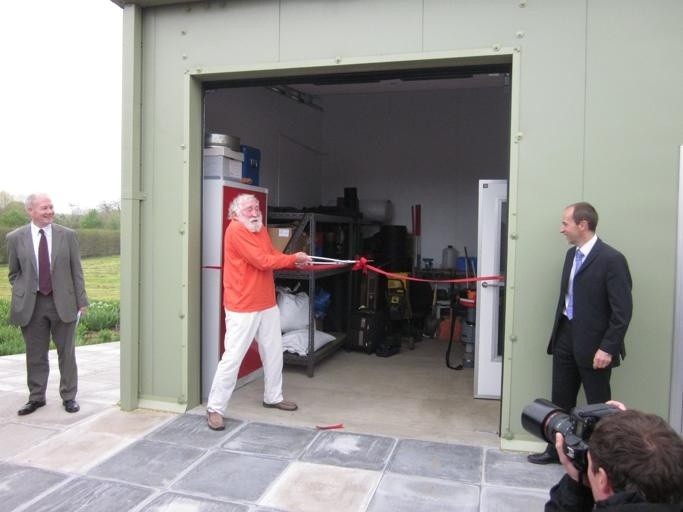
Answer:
[[415, 267, 477, 301]]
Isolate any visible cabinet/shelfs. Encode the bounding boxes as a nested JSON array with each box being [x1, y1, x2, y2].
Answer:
[[266, 212, 381, 374]]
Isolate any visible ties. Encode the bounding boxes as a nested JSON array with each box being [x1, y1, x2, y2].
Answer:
[[565, 248, 584, 320], [35, 228, 53, 296]]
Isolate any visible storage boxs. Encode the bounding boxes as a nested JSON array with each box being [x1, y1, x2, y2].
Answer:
[[267, 224, 309, 254], [202, 147, 244, 182], [241, 144, 261, 187]]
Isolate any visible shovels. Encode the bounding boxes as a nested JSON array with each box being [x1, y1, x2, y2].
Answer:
[[423, 279, 440, 339], [439, 269, 461, 343]]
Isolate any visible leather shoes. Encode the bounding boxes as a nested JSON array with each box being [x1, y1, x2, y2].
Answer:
[[206, 409, 225, 430], [527, 450, 561, 465], [262, 400, 297, 411], [63, 399, 80, 412], [17, 400, 47, 415]]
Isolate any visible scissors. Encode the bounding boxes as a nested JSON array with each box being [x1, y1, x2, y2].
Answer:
[[294, 255, 374, 266]]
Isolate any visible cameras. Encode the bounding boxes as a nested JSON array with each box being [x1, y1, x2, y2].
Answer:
[[521, 398, 620, 473]]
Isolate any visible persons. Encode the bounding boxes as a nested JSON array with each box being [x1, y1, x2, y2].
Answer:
[[543, 400, 683, 512], [527, 203, 633, 465], [5, 192, 89, 416], [206, 193, 312, 430]]
